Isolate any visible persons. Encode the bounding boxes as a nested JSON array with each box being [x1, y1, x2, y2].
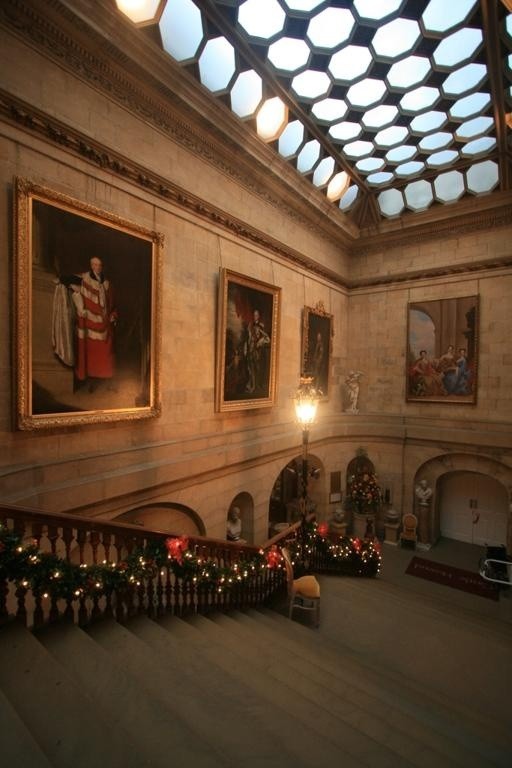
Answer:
[[228, 304, 270, 394], [227, 507, 243, 538], [311, 333, 325, 392], [413, 349, 447, 396], [442, 348, 472, 394], [435, 345, 456, 377], [73, 255, 121, 393], [415, 479, 432, 501]]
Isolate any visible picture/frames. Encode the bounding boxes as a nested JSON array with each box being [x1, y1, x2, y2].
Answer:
[[301, 300, 335, 404], [214, 265, 283, 415], [12, 172, 167, 434], [404, 292, 481, 405]]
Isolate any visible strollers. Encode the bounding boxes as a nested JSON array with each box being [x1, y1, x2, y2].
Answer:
[[478, 543, 512, 591]]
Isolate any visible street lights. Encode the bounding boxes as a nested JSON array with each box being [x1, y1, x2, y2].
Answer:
[[293, 362, 325, 580]]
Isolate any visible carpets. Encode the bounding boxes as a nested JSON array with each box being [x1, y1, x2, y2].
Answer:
[[403, 555, 500, 601]]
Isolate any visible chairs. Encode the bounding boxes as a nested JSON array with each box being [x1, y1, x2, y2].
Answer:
[[281, 547, 321, 630], [399, 512, 418, 549]]
